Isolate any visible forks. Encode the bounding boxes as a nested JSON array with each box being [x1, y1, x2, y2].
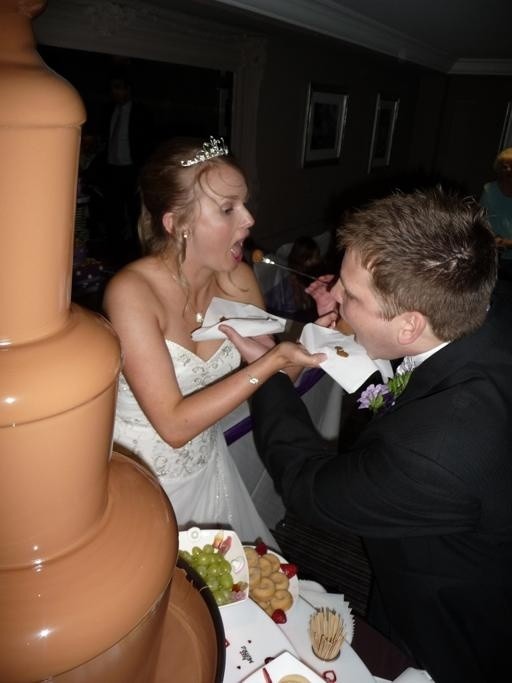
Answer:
[[260, 257, 328, 288]]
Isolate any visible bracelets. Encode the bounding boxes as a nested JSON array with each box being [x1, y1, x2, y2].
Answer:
[[240, 367, 266, 389]]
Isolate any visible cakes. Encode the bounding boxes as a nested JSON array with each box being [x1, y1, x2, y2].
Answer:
[[0, 0, 220, 682]]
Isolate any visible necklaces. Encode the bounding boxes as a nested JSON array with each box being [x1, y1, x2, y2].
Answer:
[[159, 254, 215, 324]]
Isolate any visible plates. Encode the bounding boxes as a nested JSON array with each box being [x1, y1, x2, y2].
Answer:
[[174, 526, 330, 683]]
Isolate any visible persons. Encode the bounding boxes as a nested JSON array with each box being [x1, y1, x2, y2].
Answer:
[[219, 186, 512, 678], [288, 237, 327, 284], [102, 135, 338, 552], [477, 147, 512, 284]]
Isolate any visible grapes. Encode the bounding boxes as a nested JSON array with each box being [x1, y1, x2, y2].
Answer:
[[181, 545, 234, 603]]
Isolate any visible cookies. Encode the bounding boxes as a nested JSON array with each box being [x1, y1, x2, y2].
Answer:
[[335, 317, 357, 340], [245, 546, 293, 615]]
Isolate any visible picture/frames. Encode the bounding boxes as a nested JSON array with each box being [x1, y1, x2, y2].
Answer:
[[366, 91, 400, 175], [301, 81, 350, 167]]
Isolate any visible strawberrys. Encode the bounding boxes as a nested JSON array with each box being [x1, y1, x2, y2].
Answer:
[[272, 610, 286, 623], [280, 564, 299, 580], [254, 543, 267, 554]]
